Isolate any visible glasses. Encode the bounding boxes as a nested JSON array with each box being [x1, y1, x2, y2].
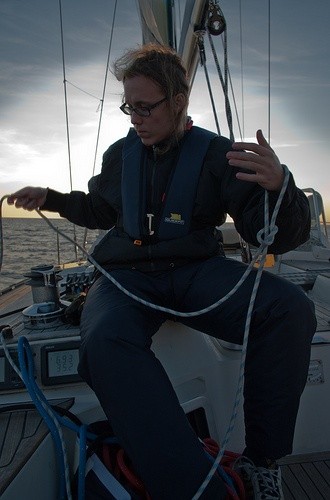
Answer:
[[119, 93, 168, 117]]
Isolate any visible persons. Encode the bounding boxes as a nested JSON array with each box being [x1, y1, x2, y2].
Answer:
[[7, 42, 316, 500]]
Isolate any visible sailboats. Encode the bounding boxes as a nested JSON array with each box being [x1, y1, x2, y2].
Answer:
[[1, 1, 329, 499]]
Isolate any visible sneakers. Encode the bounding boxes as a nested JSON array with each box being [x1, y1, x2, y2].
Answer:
[[231, 451, 283, 499]]
